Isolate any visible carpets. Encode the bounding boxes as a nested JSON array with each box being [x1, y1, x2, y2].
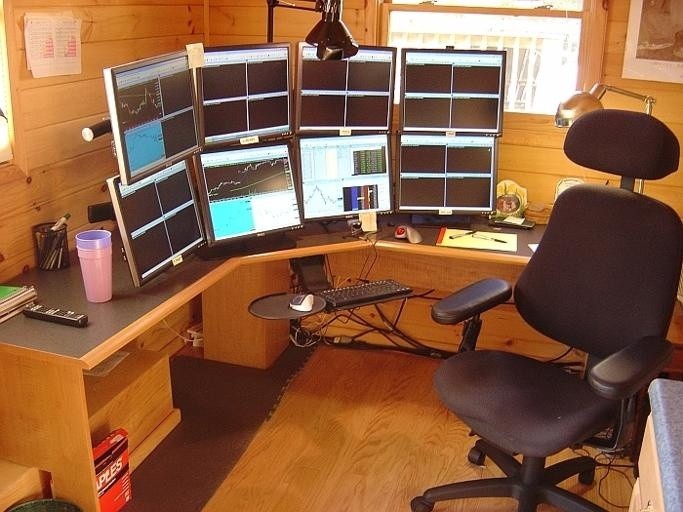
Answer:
[[118, 337, 319, 512]]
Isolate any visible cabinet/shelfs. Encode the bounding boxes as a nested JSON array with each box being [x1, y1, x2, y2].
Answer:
[[629, 379, 683, 512]]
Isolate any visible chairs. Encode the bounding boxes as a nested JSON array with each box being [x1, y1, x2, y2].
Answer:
[[411, 109, 683, 512]]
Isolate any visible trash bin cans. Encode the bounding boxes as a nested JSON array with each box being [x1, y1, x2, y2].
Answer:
[[7, 498, 82, 512]]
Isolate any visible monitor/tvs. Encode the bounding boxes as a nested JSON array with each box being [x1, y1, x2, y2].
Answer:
[[195, 41, 295, 149], [295, 40, 398, 136], [395, 130, 497, 230], [102, 49, 206, 186], [399, 47, 507, 136], [106, 158, 209, 289], [192, 139, 305, 256], [294, 129, 395, 233]]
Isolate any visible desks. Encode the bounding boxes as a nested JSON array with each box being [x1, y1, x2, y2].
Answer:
[[0, 215, 547, 512]]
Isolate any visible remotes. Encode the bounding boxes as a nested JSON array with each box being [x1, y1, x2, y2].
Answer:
[[22, 303, 88, 327]]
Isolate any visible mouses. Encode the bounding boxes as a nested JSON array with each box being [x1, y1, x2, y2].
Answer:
[[395, 224, 424, 244]]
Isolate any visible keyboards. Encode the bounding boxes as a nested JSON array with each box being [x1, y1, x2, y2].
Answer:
[[313, 279, 414, 307]]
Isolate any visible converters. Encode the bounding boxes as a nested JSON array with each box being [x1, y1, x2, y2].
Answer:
[[332, 335, 354, 346]]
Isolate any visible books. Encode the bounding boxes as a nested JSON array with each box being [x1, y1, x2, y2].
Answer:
[[0, 285, 38, 324]]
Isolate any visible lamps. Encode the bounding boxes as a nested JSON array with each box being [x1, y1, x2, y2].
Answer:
[[554, 84, 655, 194], [267, 0, 359, 61]]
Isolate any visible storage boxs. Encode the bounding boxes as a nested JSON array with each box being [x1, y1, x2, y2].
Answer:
[[92, 428, 132, 512]]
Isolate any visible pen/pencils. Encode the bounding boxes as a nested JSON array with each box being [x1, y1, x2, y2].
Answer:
[[38, 213, 71, 271], [449, 230, 507, 243]]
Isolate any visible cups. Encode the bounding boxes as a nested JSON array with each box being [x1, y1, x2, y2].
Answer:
[[73, 228, 114, 305]]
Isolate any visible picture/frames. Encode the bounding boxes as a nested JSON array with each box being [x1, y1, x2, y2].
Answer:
[[621, 0, 683, 84]]
